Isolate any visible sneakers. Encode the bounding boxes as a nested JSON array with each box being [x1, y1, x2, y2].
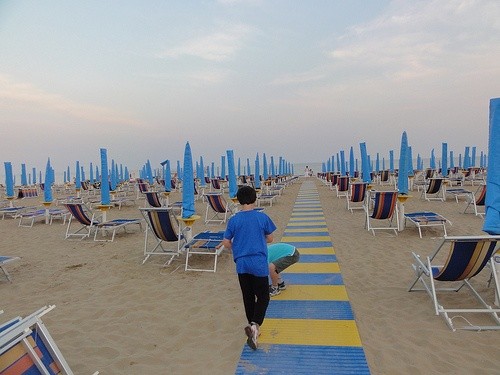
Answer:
[[269, 281, 286, 290], [244, 322, 261, 350], [269, 285, 281, 297]]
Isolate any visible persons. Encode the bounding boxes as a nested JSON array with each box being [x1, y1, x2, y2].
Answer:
[[223, 186, 277, 350], [267, 243, 299, 295], [304, 165, 313, 182]]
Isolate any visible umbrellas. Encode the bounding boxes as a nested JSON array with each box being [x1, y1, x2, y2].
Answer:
[[4, 142, 294, 257], [322, 131, 487, 231], [482, 97, 500, 306]]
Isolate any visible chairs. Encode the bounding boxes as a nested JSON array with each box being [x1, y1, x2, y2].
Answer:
[[0, 301, 99, 375], [0, 172, 298, 282], [408, 234, 500, 332], [318, 169, 487, 239]]
